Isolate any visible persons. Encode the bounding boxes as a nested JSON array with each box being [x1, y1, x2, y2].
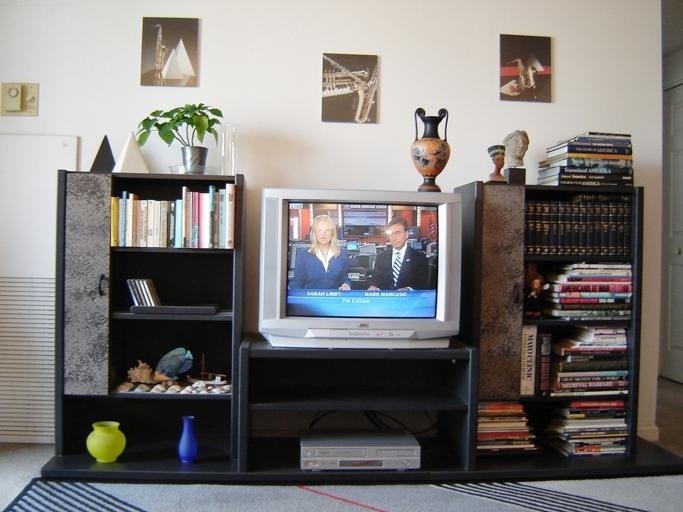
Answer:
[[484, 130, 532, 183], [368, 218, 428, 293], [294, 215, 352, 291]]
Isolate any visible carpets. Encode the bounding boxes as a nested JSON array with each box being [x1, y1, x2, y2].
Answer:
[[5, 473, 683, 510]]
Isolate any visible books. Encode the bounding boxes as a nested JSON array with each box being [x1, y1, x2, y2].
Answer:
[[520, 187, 633, 396], [126, 278, 163, 306], [111, 183, 235, 249], [477, 400, 635, 456], [537, 132, 634, 186]]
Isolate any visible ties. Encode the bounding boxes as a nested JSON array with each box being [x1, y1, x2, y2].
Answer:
[[392, 253, 402, 287]]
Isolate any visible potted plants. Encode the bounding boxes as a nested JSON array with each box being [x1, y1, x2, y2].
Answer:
[[135, 101, 223, 174]]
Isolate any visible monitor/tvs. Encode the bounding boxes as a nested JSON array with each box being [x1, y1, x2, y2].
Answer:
[[257, 185, 464, 350]]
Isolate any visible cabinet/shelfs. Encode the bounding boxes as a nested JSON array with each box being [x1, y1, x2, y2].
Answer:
[[53, 168, 244, 459], [239, 332, 471, 475], [456, 180, 644, 466]]
